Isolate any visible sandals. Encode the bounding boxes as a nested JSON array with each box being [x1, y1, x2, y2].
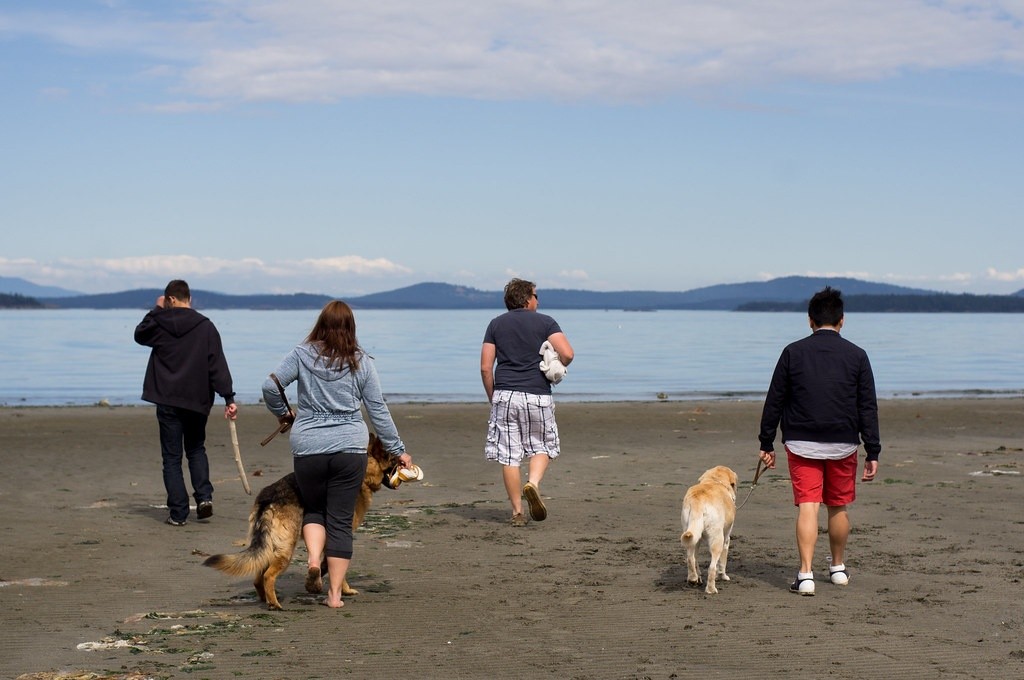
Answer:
[[387, 462, 425, 490]]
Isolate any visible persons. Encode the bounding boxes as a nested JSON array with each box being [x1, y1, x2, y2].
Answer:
[[134, 280, 238, 526], [479, 278, 574, 526], [757, 287, 882, 596], [261, 300, 413, 607]]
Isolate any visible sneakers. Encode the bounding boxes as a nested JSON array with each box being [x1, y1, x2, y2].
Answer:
[[789, 572, 820, 595], [828, 564, 851, 587]]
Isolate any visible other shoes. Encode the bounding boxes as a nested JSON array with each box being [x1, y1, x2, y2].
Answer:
[[510, 513, 525, 526], [165, 515, 187, 525], [196, 500, 215, 520], [522, 481, 548, 522]]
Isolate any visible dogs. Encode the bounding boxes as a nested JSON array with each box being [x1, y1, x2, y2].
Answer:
[[200, 431, 406, 610], [679, 464, 740, 594]]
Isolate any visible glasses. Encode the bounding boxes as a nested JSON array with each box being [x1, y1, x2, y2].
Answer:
[[532, 293, 537, 299]]
